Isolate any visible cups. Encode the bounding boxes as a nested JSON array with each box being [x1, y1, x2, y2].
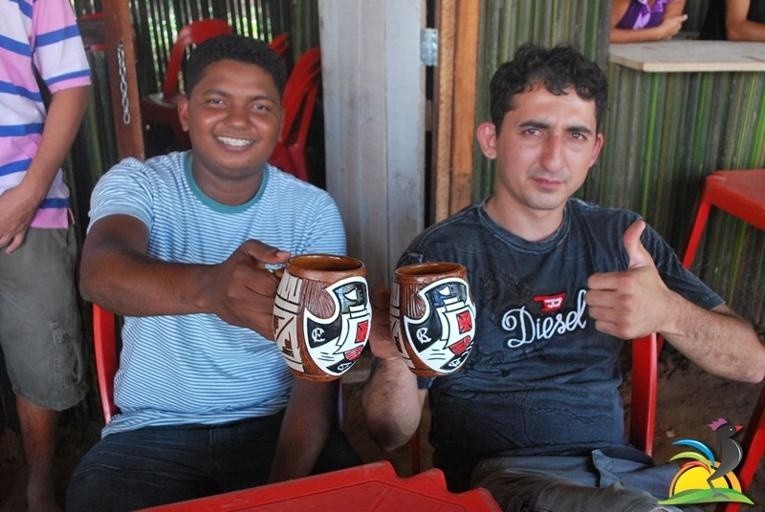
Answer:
[[388, 260, 477, 379], [270, 253, 373, 383]]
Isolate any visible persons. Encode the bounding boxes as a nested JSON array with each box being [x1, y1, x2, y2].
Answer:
[[67, 34, 352, 511], [359, 43, 764, 511], [1, 0, 93, 510], [723, 0, 765, 43], [606, 0, 688, 45]]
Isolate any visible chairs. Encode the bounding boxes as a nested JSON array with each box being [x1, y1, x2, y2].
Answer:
[[139, 23, 323, 185]]
